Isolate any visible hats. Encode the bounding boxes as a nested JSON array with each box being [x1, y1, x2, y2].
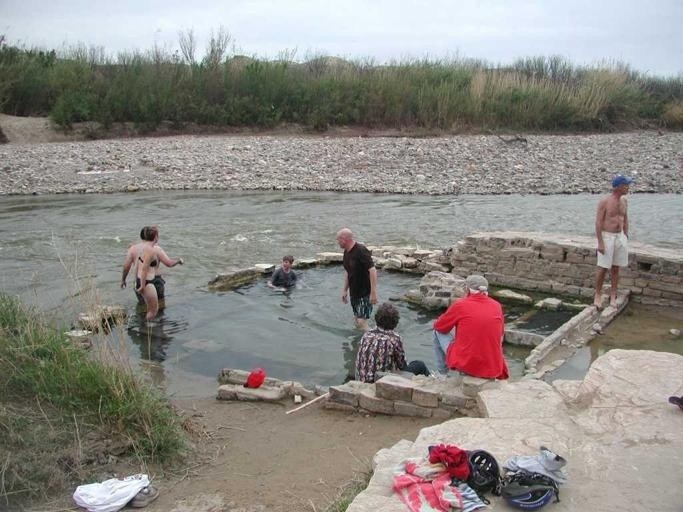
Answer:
[[463, 275, 488, 290], [611, 176, 631, 185]]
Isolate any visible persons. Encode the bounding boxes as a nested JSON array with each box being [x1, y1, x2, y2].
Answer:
[[132, 226, 158, 322], [592, 174, 629, 311], [266, 255, 296, 291], [336, 228, 377, 335], [432, 274, 504, 381], [119, 226, 183, 302], [354, 303, 430, 383]]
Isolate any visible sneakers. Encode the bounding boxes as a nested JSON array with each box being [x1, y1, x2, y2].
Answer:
[[130, 485, 158, 508]]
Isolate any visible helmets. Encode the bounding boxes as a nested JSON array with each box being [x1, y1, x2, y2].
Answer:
[[501, 473, 552, 509], [466, 451, 498, 490]]
